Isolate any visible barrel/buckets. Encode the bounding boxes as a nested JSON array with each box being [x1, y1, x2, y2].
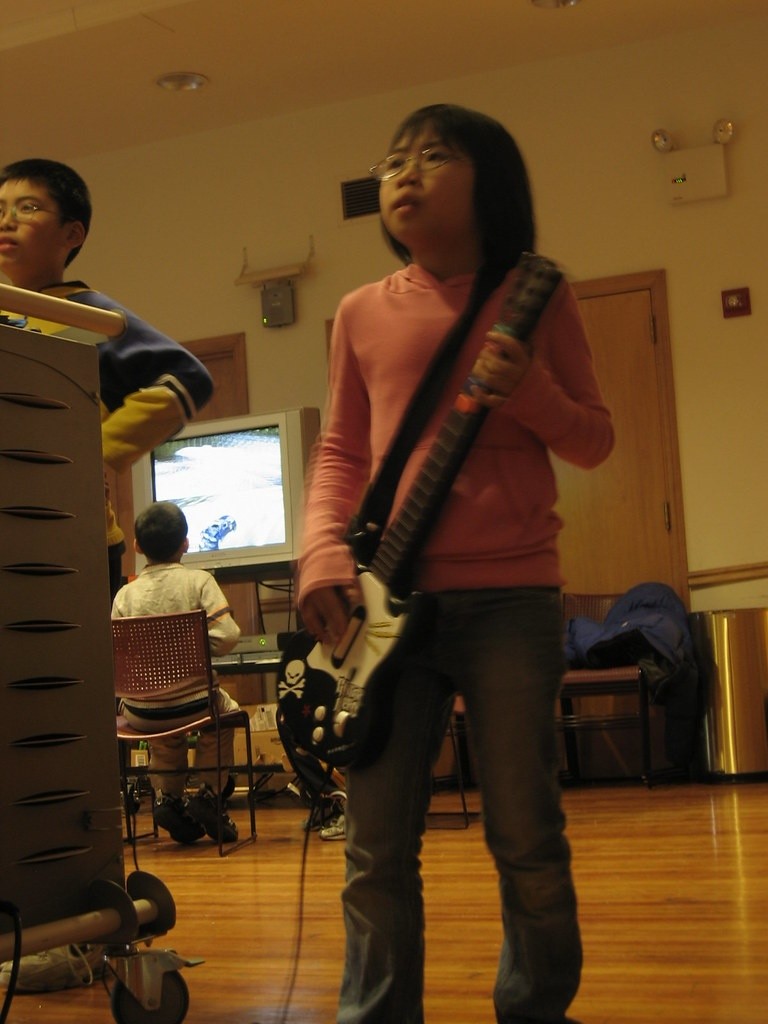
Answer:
[[686, 607, 768, 783]]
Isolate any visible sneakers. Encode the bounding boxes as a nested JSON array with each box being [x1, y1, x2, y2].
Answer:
[[0, 942, 104, 992]]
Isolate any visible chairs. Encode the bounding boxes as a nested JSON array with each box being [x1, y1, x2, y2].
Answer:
[[108, 607, 258, 856], [443, 590, 652, 830]]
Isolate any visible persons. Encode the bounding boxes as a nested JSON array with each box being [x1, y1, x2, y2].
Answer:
[[0, 160, 216, 992], [112, 501, 242, 846], [273, 563, 374, 830], [296, 104, 614, 1024]]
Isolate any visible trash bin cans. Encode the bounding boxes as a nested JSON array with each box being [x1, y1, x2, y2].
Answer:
[[686, 608, 768, 782]]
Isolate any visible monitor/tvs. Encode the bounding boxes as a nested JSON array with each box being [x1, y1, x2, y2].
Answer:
[[147, 408, 321, 571]]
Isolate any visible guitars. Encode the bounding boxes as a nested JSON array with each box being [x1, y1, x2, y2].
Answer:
[[272, 244, 565, 770]]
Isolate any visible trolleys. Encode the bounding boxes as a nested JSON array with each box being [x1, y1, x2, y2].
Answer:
[[0, 282, 207, 1024]]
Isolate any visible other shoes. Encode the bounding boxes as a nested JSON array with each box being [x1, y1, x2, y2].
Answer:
[[153, 792, 207, 844], [319, 815, 347, 841], [185, 783, 239, 843]]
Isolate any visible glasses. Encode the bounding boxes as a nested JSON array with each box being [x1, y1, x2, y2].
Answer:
[[368, 144, 457, 181], [0, 201, 60, 224]]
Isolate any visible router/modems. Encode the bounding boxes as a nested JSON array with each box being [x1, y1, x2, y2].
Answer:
[[259, 280, 295, 327]]
